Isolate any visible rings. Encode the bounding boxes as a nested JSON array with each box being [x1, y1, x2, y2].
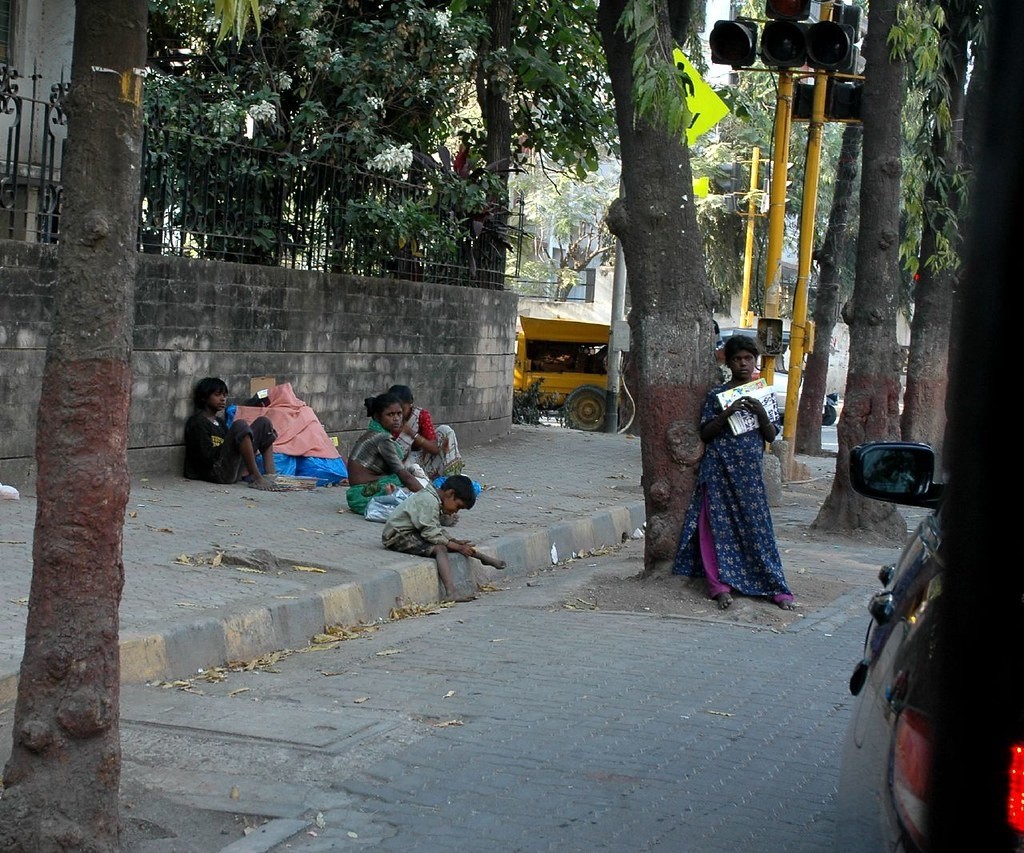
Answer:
[[750, 403, 754, 409]]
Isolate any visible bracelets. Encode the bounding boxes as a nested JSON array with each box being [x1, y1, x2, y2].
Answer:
[[413, 433, 426, 446]]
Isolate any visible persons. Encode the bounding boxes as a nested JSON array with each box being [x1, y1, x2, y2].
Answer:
[[382, 474, 509, 603], [671, 336, 798, 613], [346, 386, 461, 527], [184, 377, 282, 492]]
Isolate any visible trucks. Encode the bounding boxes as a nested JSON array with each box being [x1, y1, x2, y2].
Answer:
[[510, 312, 626, 435]]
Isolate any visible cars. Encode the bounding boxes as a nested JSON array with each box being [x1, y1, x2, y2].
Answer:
[[717, 328, 827, 427], [842, 437, 1024, 852]]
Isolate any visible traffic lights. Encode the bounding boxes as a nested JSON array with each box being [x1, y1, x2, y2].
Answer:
[[791, 0, 866, 124], [709, 0, 854, 74]]
